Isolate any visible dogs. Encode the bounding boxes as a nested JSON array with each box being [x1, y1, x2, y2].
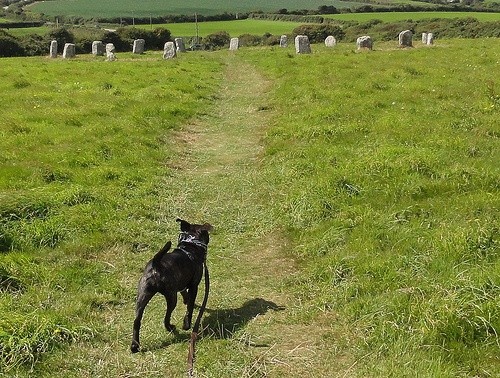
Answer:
[[130, 217, 215, 354]]
